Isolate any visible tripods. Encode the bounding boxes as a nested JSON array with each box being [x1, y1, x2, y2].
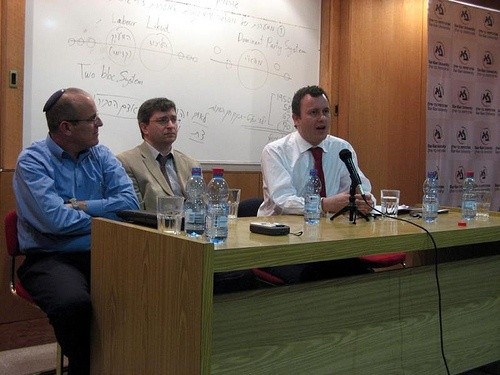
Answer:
[[330, 182, 369, 225]]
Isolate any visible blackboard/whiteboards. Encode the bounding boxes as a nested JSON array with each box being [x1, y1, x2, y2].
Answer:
[[21, 1, 326, 176]]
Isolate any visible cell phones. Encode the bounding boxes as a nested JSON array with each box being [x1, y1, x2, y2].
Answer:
[[250, 223, 290, 236]]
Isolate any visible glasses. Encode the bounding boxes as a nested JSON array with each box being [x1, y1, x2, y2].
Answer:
[[144, 118, 181, 126], [59, 112, 98, 124]]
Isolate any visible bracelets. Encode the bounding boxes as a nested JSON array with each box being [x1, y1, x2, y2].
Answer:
[[321, 198, 326, 213]]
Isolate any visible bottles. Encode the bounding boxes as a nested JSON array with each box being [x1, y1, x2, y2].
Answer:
[[204, 168, 229, 244], [422, 170, 439, 223], [462, 171, 477, 221], [184, 167, 206, 238], [304, 168, 322, 224]]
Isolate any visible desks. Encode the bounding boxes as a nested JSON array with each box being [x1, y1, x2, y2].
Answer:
[[87, 207, 500, 375]]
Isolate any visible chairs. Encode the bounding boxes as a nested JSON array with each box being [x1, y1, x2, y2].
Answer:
[[354, 253, 408, 272], [238, 198, 284, 286], [5, 210, 64, 375]]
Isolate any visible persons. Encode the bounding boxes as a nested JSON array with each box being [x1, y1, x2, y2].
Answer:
[[14, 87, 141, 375], [116, 98, 203, 212], [257, 86, 376, 285]]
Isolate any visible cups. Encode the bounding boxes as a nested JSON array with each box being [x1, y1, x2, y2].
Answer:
[[381, 189, 400, 218], [474, 192, 491, 221], [156, 195, 185, 235], [219, 188, 240, 221]]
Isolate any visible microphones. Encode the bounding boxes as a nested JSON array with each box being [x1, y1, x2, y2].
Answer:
[[338, 148, 365, 199]]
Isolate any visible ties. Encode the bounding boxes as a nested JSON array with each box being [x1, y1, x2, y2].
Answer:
[[307, 147, 326, 199]]
